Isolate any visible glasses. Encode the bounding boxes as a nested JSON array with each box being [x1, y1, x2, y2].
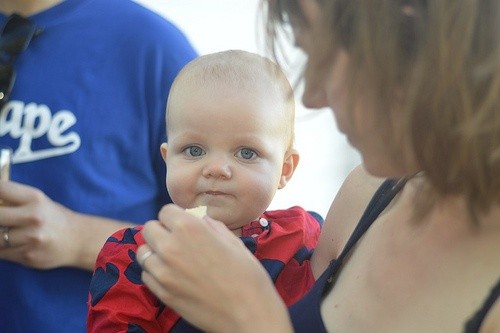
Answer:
[[0, 12, 45, 107]]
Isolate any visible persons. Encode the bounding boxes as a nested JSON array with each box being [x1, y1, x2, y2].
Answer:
[[85, 51, 323, 333], [1, 0, 200, 332], [136, 0, 500, 333]]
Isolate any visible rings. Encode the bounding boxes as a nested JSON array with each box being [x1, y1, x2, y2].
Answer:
[[138, 249, 153, 265], [4, 229, 11, 248]]
